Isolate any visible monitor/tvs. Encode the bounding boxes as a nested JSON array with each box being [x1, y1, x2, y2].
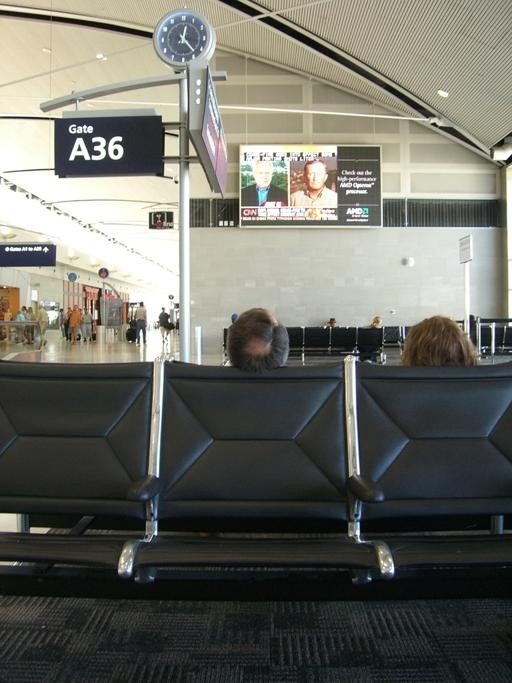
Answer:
[[237, 143, 381, 229]]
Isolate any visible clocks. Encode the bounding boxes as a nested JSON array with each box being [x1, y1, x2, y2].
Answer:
[[152, 9, 213, 66]]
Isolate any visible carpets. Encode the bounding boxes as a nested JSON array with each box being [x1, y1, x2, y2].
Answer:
[[0, 525, 512, 682]]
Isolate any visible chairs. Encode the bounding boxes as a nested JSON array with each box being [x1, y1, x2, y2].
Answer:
[[222, 314, 512, 364], [125, 360, 394, 598], [346, 356, 510, 598], [2, 356, 160, 600]]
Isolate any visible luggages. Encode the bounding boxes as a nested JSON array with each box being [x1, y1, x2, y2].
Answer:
[[126, 323, 136, 341]]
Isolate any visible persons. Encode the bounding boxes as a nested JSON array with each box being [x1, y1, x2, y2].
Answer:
[[127, 302, 169, 345], [15, 303, 49, 349], [243, 156, 339, 207], [401, 314, 481, 367], [226, 308, 290, 373], [56, 303, 93, 345]]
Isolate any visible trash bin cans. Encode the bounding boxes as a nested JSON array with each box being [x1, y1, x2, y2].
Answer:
[[192, 327, 202, 359]]
